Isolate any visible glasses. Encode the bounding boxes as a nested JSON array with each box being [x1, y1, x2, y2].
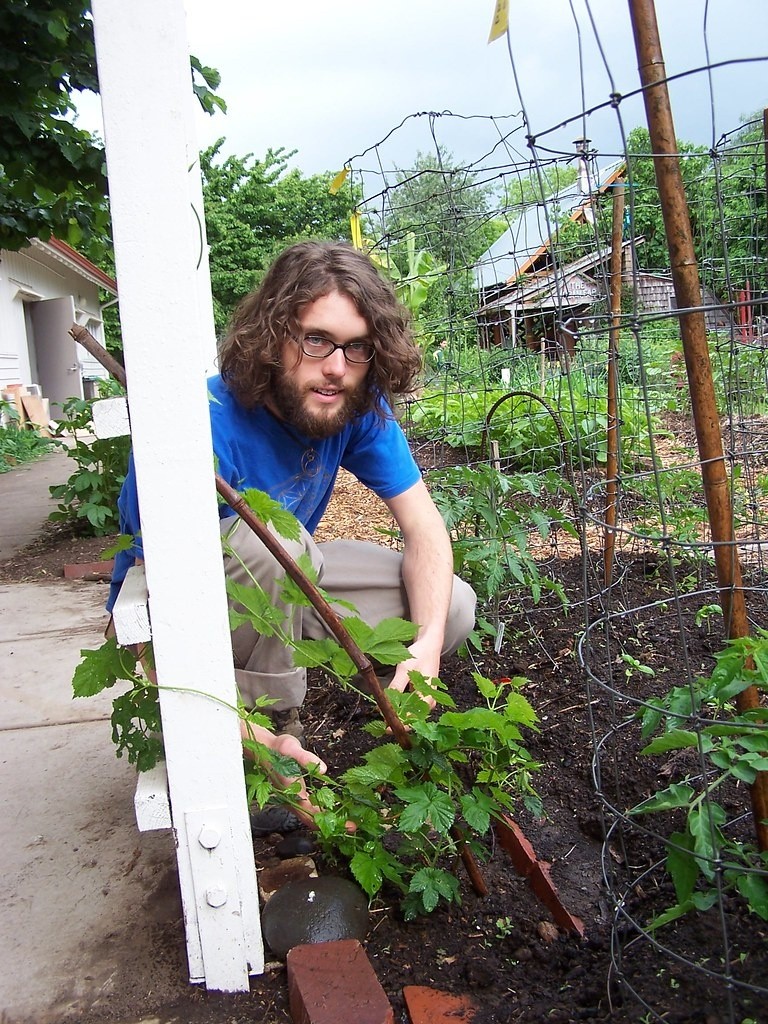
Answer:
[[274, 320, 375, 363]]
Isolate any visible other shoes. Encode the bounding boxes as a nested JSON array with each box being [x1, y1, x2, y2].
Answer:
[[246, 797, 303, 837], [244, 706, 308, 751]]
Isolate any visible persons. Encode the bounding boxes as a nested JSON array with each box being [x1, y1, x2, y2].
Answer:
[[105, 238, 476, 839], [433, 339, 447, 370]]
